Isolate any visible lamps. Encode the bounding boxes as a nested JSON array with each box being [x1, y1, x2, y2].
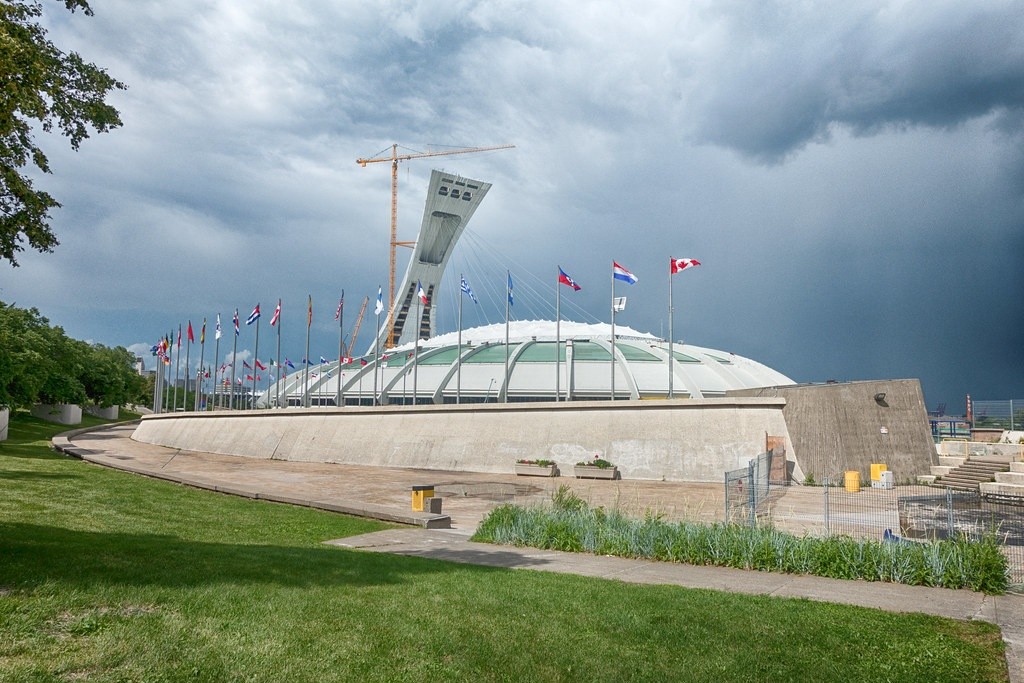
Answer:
[[874, 393, 885, 401]]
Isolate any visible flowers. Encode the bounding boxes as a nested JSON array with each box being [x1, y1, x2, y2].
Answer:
[[577, 454, 613, 470], [516, 459, 556, 467]]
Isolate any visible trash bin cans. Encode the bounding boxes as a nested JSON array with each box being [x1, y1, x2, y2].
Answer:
[[845, 470, 860, 493]]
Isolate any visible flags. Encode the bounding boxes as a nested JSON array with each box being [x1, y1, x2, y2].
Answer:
[[557, 265, 581, 292], [613, 262, 638, 285], [670, 257, 701, 275], [199, 350, 414, 387], [506, 273, 514, 305], [375, 288, 384, 314], [460, 276, 477, 304], [150, 288, 345, 366], [417, 281, 429, 305]]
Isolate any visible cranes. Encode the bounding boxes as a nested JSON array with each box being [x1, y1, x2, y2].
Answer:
[[356, 143, 515, 349], [341, 296, 370, 358]]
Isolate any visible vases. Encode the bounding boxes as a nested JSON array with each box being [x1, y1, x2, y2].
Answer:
[[516, 464, 557, 476], [574, 465, 617, 478]]
